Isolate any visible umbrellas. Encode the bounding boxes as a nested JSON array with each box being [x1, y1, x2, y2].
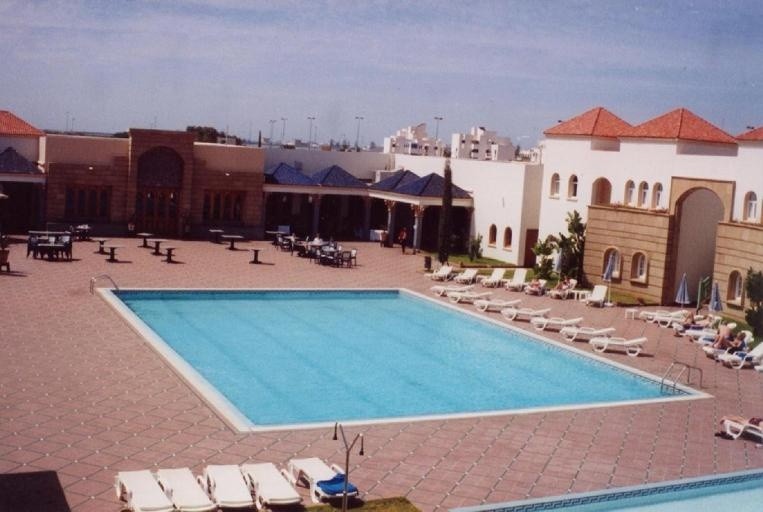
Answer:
[[602, 254, 615, 303], [675, 271, 692, 308], [708, 279, 724, 313]]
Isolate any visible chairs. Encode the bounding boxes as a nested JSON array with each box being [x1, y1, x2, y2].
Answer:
[[280, 233, 357, 268], [0, 226, 90, 273], [114, 457, 358, 512], [721, 419, 763, 439]]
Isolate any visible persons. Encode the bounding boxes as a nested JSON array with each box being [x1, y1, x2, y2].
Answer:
[[554, 275, 572, 290], [313, 233, 324, 258], [379, 228, 387, 247], [397, 226, 408, 253], [682, 312, 714, 326], [524, 275, 541, 295], [712, 331, 747, 353], [716, 320, 734, 341]]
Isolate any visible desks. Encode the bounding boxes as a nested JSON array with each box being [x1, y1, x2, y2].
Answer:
[[90, 229, 284, 265]]
